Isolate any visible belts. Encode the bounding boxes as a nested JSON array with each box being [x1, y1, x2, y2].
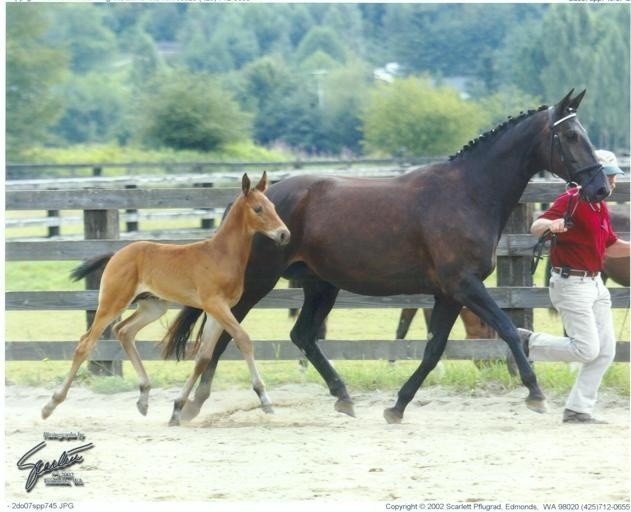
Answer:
[[551, 267, 600, 277]]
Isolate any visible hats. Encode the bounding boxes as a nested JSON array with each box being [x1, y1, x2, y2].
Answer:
[[593, 150, 625, 176]]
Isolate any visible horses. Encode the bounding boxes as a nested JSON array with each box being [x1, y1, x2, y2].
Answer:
[[41, 170, 291, 428], [545, 219, 630, 337], [152, 87, 612, 425], [285, 281, 498, 378]]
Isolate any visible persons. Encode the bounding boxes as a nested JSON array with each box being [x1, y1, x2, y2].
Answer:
[[507, 149, 630, 424]]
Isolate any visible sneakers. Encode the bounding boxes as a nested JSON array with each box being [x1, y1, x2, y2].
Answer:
[[507, 328, 532, 377], [562, 410, 607, 424]]
